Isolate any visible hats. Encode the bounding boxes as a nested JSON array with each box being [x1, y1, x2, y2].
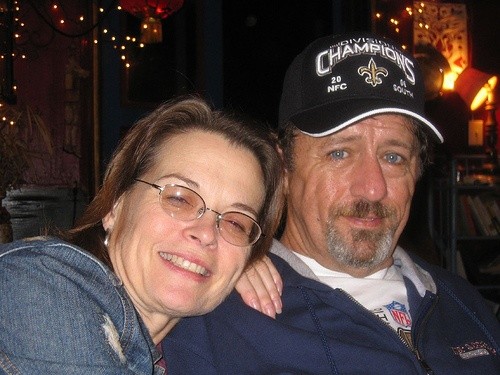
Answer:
[[279, 32, 444, 143]]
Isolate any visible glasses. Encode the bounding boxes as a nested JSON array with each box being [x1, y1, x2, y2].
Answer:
[[133, 179, 266, 247]]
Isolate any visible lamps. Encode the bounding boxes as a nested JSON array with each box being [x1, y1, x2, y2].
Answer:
[[454, 66, 497, 147]]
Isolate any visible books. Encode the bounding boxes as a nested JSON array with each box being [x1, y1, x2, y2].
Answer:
[[456, 193, 500, 237]]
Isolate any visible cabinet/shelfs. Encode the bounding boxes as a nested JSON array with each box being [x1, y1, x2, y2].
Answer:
[[422, 156, 500, 289]]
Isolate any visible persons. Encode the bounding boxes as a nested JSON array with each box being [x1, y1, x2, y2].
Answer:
[[160, 30, 500, 375], [0, 94, 285, 375]]
